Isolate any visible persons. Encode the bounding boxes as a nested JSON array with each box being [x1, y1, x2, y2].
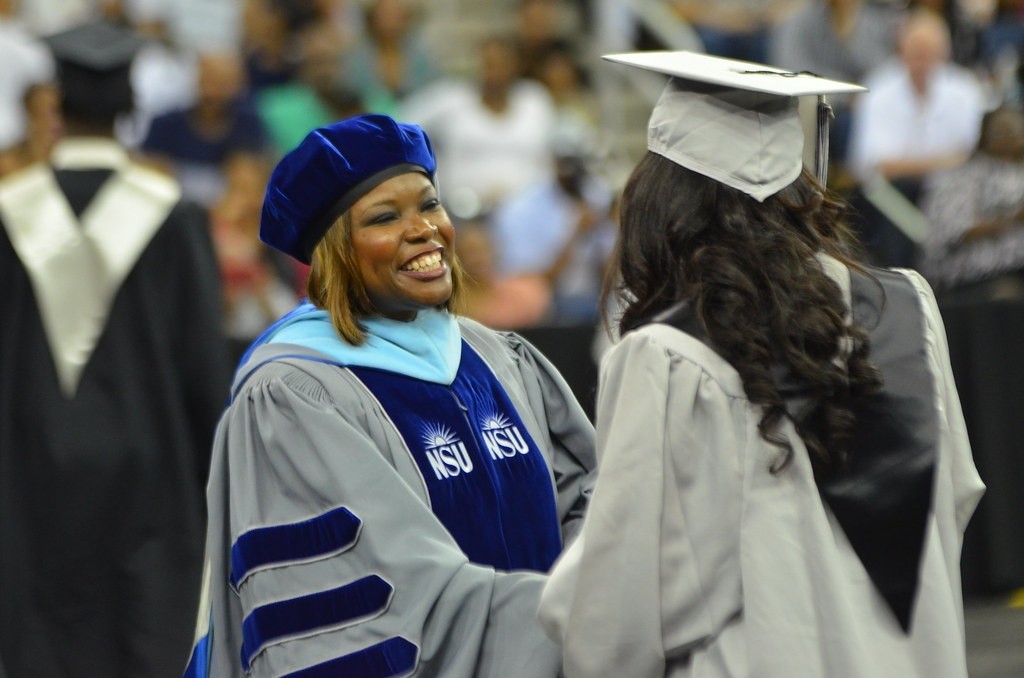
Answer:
[[183, 117, 595, 678], [0, 23, 233, 678], [0, 1, 1023, 609], [535, 50, 987, 678]]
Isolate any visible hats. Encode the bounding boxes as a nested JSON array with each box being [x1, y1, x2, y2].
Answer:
[[44, 23, 147, 112], [603, 51, 871, 200], [258, 114, 438, 266]]
